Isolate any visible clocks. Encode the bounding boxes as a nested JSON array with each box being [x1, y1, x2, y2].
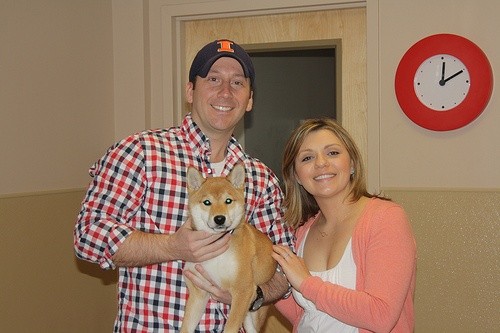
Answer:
[[394, 34, 494, 131]]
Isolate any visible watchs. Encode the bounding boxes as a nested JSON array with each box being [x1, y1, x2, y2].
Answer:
[[248, 285, 264, 312]]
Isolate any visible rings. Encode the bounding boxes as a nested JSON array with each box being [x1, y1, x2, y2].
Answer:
[[283, 254, 290, 259]]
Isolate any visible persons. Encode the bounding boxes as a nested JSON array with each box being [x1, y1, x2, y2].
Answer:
[[272, 118, 416, 333], [73, 39, 296, 333]]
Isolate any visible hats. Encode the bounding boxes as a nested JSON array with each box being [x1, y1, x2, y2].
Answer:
[[189, 39, 254, 77]]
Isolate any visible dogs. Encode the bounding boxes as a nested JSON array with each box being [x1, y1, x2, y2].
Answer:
[[178, 163, 278, 333]]
